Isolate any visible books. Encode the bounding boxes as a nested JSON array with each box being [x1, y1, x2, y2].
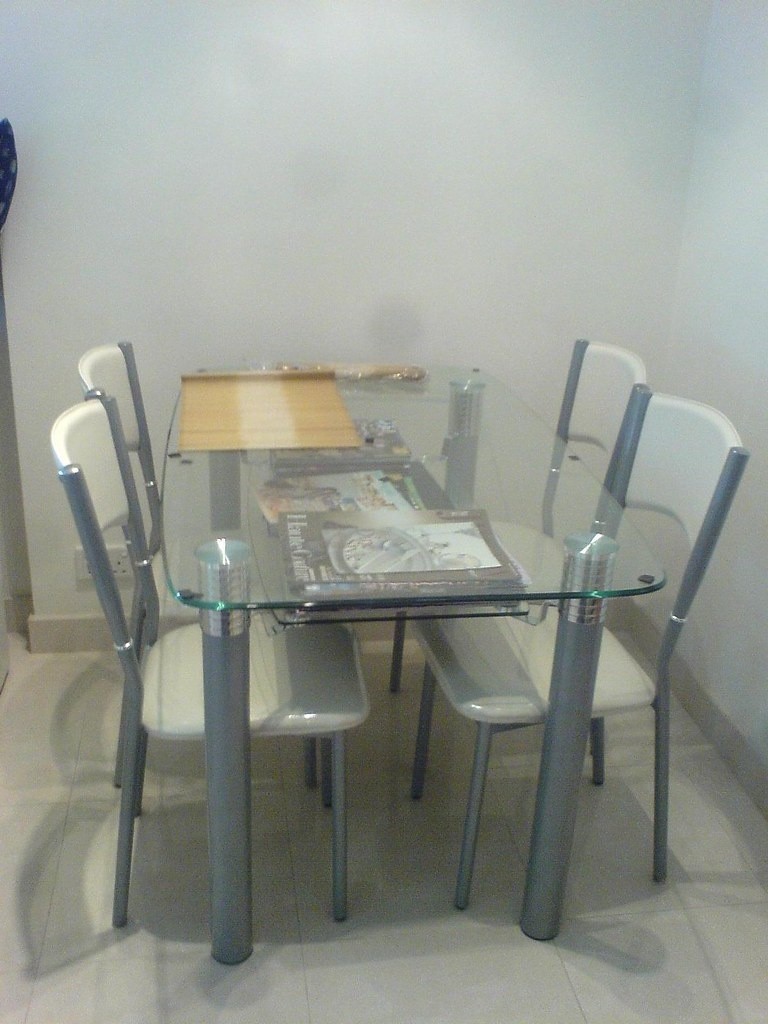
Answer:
[[257, 411, 532, 615]]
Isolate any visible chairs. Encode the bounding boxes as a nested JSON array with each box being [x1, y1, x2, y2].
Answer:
[[409, 386, 749, 910], [389, 341, 651, 755], [76, 341, 318, 796], [51, 397, 369, 930]]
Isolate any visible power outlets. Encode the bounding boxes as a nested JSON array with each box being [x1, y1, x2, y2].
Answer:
[[72, 544, 135, 578]]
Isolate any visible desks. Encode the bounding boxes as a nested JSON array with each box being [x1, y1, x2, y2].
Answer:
[[158, 367, 668, 964]]
